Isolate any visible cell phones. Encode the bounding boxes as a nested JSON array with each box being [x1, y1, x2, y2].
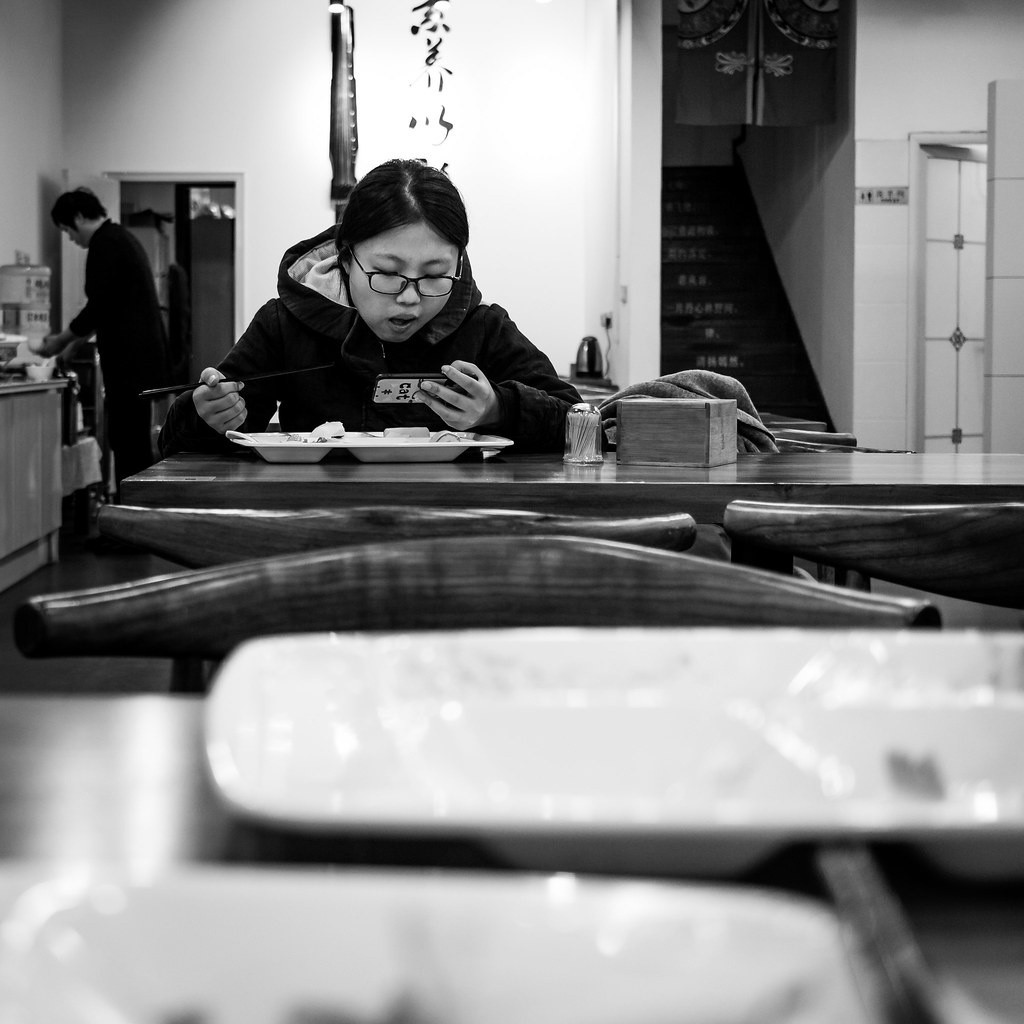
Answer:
[[372, 373, 479, 404]]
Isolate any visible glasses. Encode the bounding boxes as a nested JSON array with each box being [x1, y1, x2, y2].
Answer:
[[346, 241, 464, 297]]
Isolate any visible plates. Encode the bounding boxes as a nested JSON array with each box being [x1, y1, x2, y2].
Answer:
[[197, 627, 1024, 843], [224, 431, 514, 463], [0, 857, 875, 1024]]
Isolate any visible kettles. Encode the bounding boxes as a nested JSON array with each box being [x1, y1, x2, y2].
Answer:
[[575, 335, 604, 379]]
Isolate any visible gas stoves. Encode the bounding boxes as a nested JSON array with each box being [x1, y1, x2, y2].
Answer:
[[0, 362, 69, 386]]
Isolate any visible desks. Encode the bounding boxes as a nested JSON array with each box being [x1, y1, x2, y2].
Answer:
[[0, 688, 1024, 1024], [121, 451, 1024, 587]]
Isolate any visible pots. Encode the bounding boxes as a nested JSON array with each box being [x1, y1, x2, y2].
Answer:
[[0, 337, 28, 361]]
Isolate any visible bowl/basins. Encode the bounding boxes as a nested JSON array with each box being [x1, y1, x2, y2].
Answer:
[[25, 366, 52, 382], [28, 340, 42, 352]]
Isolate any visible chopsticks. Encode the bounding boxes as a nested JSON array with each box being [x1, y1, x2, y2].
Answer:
[[138, 362, 338, 397]]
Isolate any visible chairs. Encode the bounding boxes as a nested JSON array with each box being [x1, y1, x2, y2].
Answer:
[[14, 499, 1024, 688]]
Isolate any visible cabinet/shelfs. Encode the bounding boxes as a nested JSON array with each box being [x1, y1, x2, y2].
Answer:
[[0, 379, 71, 597]]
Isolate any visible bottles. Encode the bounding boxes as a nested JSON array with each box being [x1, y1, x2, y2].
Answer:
[[0, 265, 52, 336]]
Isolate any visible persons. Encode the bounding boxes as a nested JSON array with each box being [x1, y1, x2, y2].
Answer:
[[157, 158, 607, 460], [38, 186, 168, 559]]
